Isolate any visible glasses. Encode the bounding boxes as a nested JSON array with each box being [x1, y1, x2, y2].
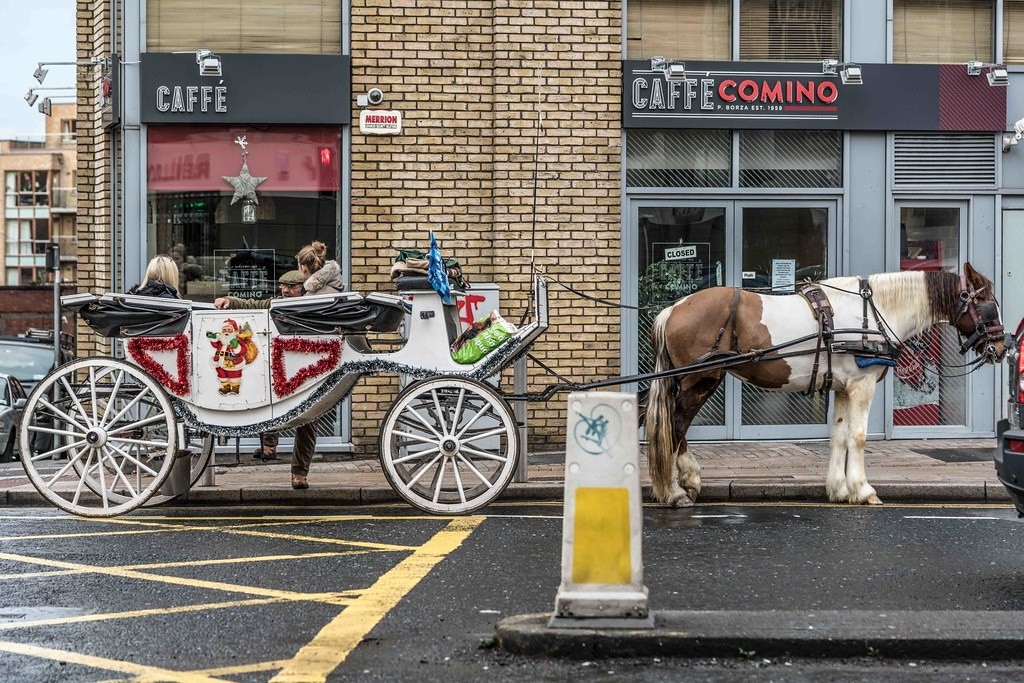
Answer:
[[279, 283, 299, 289]]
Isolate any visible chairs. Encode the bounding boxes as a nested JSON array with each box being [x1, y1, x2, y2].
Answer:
[[56, 292, 191, 380], [270, 294, 411, 404]]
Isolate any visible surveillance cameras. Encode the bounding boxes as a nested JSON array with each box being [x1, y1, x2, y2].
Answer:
[[367, 88, 384, 105]]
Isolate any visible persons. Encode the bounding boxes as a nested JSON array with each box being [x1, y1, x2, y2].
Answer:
[[297, 241, 345, 297], [166, 244, 201, 294], [214, 270, 312, 490], [125, 255, 181, 300]]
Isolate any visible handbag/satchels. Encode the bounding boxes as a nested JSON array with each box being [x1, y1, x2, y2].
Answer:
[[450, 309, 518, 364]]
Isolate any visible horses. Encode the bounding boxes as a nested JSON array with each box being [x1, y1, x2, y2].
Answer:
[[644, 261, 1007, 508]]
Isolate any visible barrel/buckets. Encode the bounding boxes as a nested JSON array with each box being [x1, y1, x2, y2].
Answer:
[[147, 450, 192, 496]]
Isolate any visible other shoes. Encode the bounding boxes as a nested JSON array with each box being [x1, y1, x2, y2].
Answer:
[[292, 474, 309, 489], [253, 446, 276, 459]]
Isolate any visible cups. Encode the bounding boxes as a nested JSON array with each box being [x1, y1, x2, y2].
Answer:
[[894, 342, 926, 390]]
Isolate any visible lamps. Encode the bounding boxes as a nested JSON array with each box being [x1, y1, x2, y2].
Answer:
[[1002, 118, 1024, 153], [34, 60, 105, 82], [196, 49, 221, 78], [822, 56, 862, 86], [969, 60, 1007, 88], [651, 56, 687, 85], [38, 99, 78, 116], [23, 85, 77, 106]]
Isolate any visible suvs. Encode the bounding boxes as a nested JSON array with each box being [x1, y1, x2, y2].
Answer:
[[0, 329, 74, 459]]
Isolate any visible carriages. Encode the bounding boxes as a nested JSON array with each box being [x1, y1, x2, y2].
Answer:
[[19, 113, 1008, 516]]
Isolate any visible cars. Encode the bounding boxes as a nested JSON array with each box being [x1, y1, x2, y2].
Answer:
[[994, 318, 1024, 518], [0, 373, 37, 463]]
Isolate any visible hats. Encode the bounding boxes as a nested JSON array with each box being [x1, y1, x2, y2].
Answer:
[[278, 270, 307, 283]]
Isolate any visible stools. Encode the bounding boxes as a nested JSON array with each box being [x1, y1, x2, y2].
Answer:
[[398, 274, 461, 292]]
